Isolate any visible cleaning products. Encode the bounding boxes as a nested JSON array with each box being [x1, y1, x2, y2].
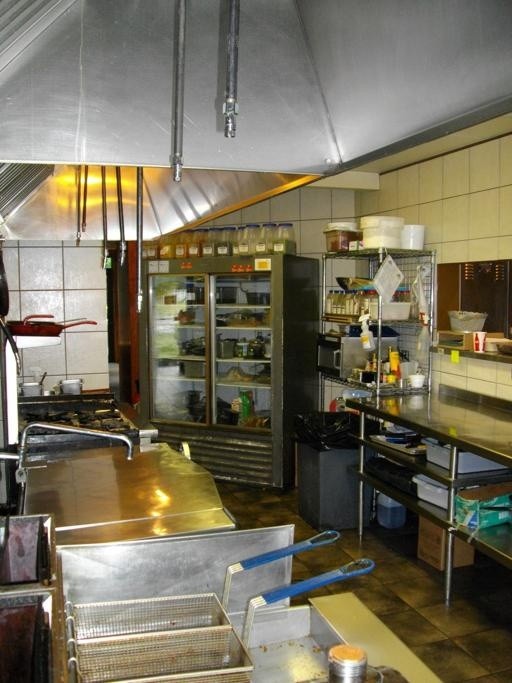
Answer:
[[415, 312, 431, 354], [357, 314, 376, 352]]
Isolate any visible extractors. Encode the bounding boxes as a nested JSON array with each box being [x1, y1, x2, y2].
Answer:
[[3, 0, 82, 75], [0, 161, 58, 221]]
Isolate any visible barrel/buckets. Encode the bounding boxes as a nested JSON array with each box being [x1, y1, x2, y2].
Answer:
[[376, 492, 407, 531], [141, 222, 297, 261], [401, 224, 425, 252]]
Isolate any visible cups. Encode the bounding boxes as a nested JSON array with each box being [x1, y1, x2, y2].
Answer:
[[471, 330, 487, 353], [400, 224, 424, 250], [409, 373, 425, 387], [406, 394, 423, 410]]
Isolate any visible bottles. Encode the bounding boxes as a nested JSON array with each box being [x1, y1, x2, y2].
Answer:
[[263, 333, 271, 358], [238, 389, 251, 417], [325, 641, 369, 683], [389, 351, 401, 378], [324, 288, 413, 321], [364, 357, 377, 372], [184, 282, 195, 303], [386, 399, 399, 417], [257, 331, 264, 341], [351, 367, 363, 380]]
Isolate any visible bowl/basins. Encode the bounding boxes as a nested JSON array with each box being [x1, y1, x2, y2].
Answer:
[[245, 291, 270, 304], [496, 339, 512, 355], [448, 309, 489, 332], [484, 336, 511, 352]]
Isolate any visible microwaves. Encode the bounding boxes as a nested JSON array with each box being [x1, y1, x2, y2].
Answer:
[[315, 332, 399, 378]]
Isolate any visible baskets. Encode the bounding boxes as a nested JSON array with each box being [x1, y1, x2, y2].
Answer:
[[448, 311, 488, 334]]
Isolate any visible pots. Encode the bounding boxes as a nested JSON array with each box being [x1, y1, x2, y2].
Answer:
[[6, 314, 98, 336], [20, 371, 49, 400]]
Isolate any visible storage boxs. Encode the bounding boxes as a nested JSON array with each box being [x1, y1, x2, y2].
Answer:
[[413, 511, 474, 572]]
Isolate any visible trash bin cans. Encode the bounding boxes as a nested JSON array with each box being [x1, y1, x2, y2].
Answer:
[[289, 411, 380, 533]]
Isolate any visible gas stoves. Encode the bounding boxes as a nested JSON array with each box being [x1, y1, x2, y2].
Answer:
[[23, 403, 136, 436]]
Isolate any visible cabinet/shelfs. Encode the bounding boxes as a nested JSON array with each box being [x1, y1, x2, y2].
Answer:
[[343, 389, 510, 609], [151, 300, 270, 390], [317, 245, 432, 418]]
